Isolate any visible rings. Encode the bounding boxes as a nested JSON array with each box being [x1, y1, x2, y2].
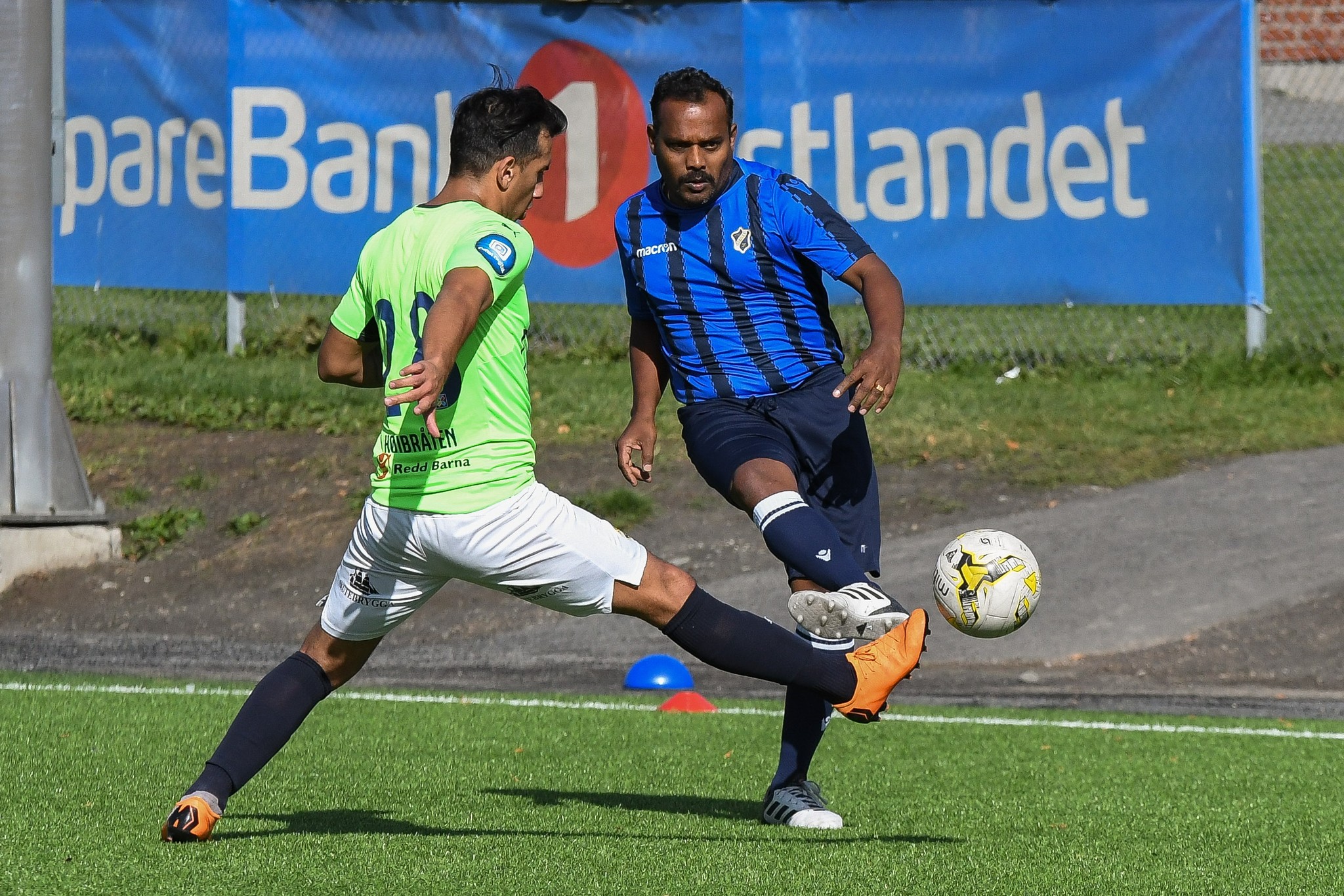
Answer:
[[874, 384, 884, 392]]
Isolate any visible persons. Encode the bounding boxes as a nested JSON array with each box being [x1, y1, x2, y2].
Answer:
[[157, 63, 933, 842], [614, 67, 904, 830]]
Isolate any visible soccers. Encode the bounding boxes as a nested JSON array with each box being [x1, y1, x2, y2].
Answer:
[[933, 529, 1042, 639]]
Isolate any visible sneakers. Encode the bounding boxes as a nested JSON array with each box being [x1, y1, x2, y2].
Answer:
[[158, 797, 221, 844], [760, 779, 844, 832], [787, 580, 911, 641], [832, 608, 931, 725]]
